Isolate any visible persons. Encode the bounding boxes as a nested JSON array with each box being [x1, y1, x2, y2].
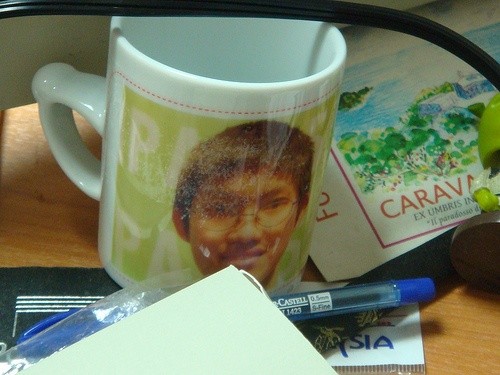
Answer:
[[171, 118, 316, 289]]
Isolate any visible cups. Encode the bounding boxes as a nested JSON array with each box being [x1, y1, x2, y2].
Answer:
[[31, 16, 348, 298]]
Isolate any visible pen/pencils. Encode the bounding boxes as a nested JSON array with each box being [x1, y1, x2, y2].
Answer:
[[269, 276, 438, 323]]
